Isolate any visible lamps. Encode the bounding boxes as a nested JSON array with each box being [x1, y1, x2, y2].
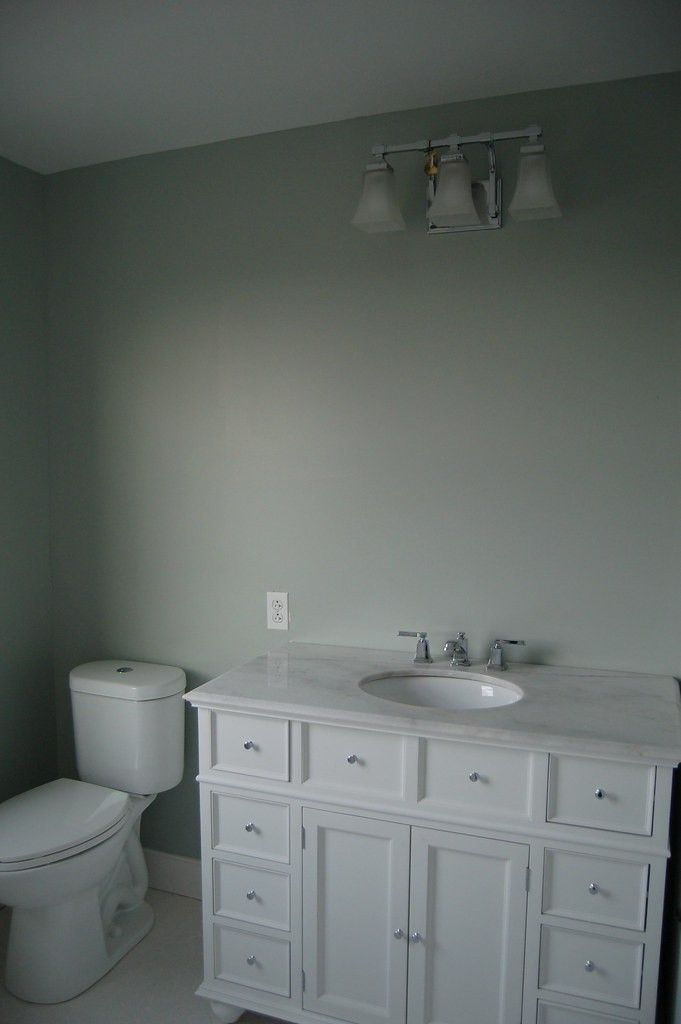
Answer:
[[349, 122, 564, 234]]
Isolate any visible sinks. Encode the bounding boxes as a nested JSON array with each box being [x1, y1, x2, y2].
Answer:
[[357, 666, 524, 714]]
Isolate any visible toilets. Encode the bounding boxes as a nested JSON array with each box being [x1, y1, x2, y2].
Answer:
[[0, 654, 189, 1010]]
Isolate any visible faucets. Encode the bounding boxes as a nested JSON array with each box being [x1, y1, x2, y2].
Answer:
[[442, 631, 473, 669]]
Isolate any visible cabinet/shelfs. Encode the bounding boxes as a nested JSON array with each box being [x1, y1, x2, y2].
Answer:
[[181, 690, 681, 1024]]
